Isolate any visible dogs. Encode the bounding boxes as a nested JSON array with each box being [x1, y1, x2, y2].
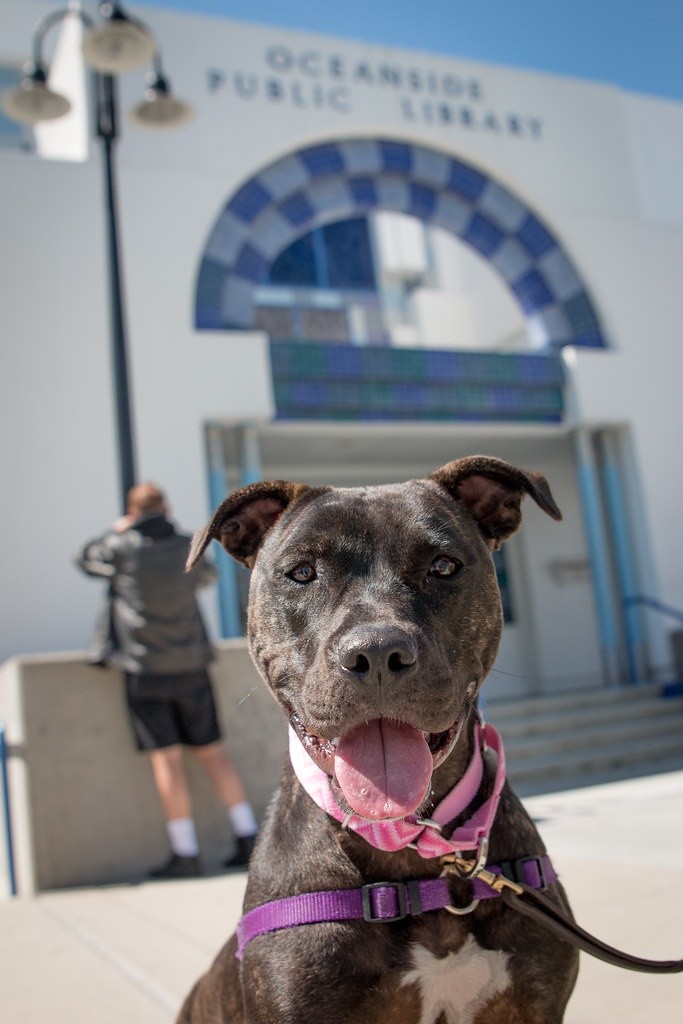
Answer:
[[173, 455, 582, 1024]]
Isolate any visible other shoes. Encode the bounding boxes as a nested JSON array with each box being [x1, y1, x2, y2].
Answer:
[[223, 834, 260, 868], [146, 854, 201, 878]]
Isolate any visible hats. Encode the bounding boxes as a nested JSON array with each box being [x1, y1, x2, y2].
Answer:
[[113, 484, 168, 534]]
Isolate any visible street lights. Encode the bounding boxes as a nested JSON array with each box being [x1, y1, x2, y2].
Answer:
[[1, 0, 192, 651]]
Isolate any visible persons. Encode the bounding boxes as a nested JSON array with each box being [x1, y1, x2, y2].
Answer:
[[78, 481, 258, 879]]
